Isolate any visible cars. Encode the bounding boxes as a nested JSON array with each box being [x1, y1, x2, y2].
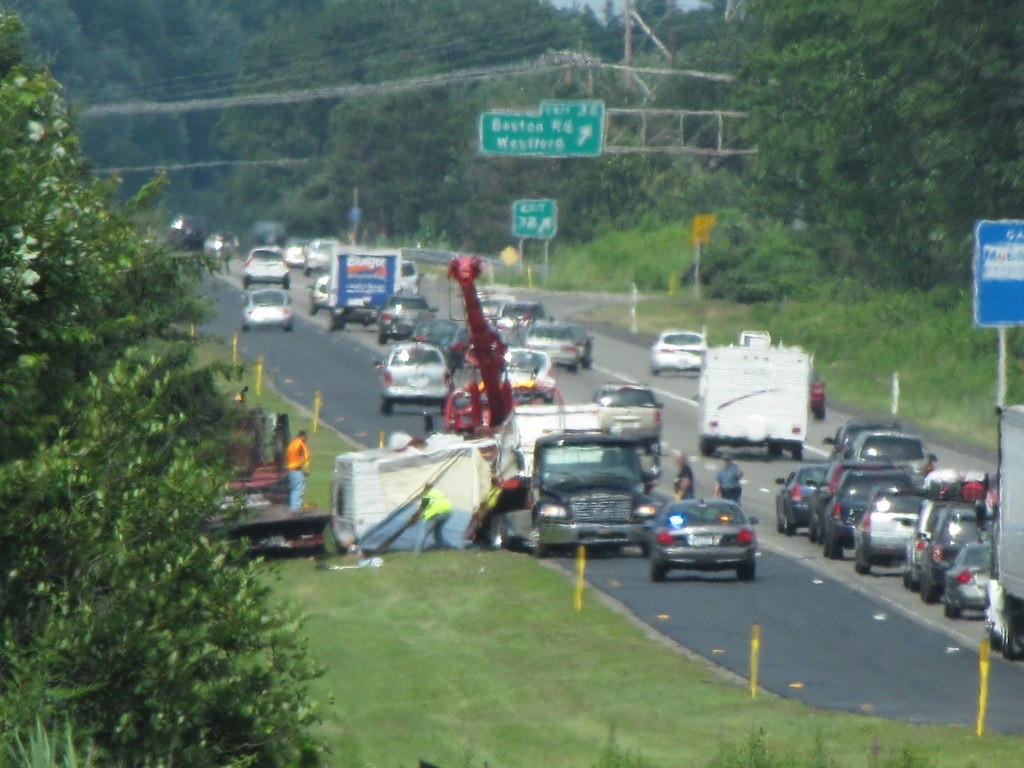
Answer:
[[922, 469, 991, 501], [170, 217, 342, 331], [808, 372, 827, 419], [850, 489, 927, 572], [377, 292, 706, 443], [944, 544, 990, 616], [773, 466, 829, 535], [902, 503, 992, 604]]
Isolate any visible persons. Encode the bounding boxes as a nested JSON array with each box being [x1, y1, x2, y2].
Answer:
[[713, 452, 744, 506], [408, 482, 456, 553], [285, 429, 312, 515], [672, 450, 695, 501]]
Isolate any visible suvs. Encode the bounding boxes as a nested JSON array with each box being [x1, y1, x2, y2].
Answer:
[[809, 460, 916, 558], [401, 262, 421, 294], [822, 418, 936, 486]]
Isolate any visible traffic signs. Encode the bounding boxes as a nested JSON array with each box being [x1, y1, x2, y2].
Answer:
[[513, 200, 558, 242], [479, 97, 604, 157]]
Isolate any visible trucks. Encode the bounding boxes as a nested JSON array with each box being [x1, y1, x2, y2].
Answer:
[[976, 402, 1024, 657], [329, 246, 401, 337], [483, 434, 659, 554], [698, 331, 814, 462], [207, 412, 331, 558], [441, 251, 568, 465], [648, 498, 760, 581]]
